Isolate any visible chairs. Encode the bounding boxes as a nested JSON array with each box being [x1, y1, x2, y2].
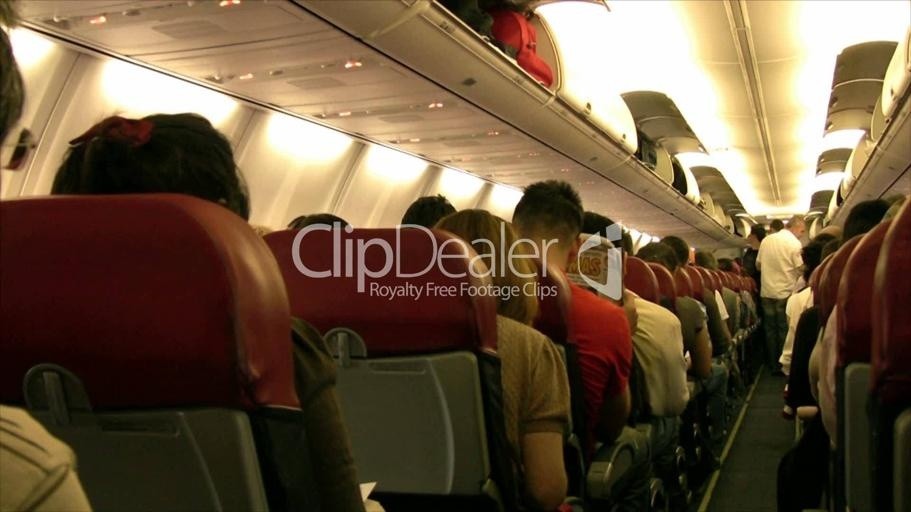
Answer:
[[794, 195, 911, 512]]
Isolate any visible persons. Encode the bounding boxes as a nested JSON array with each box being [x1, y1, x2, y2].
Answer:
[[0, 28, 911, 512]]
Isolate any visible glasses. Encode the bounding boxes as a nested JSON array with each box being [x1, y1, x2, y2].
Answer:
[[0, 129, 35, 171]]
